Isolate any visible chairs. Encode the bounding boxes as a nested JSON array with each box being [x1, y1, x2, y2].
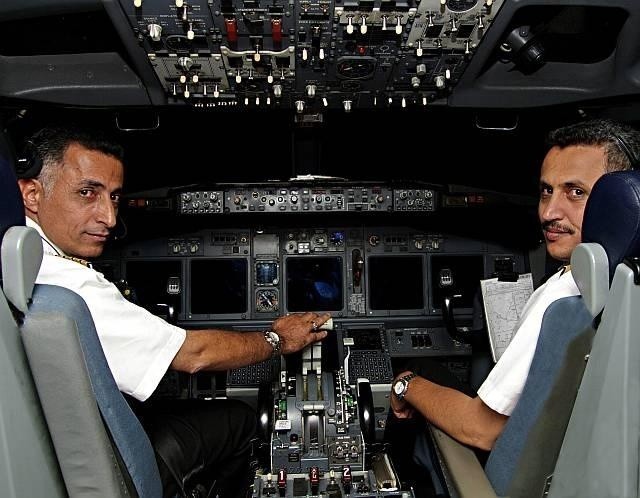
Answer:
[[0, 226, 165, 498], [426, 171, 639, 498]]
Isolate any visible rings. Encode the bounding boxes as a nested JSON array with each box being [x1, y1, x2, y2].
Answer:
[[311, 320, 318, 330]]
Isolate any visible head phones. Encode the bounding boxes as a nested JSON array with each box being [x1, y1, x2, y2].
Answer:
[[16, 125, 42, 179]]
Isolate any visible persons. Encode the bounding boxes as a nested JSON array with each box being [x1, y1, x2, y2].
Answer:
[[19, 119, 331, 497], [382, 118, 637, 497]]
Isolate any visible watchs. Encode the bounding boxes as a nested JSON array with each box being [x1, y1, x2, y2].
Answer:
[[262, 329, 282, 359], [391, 372, 417, 402]]
